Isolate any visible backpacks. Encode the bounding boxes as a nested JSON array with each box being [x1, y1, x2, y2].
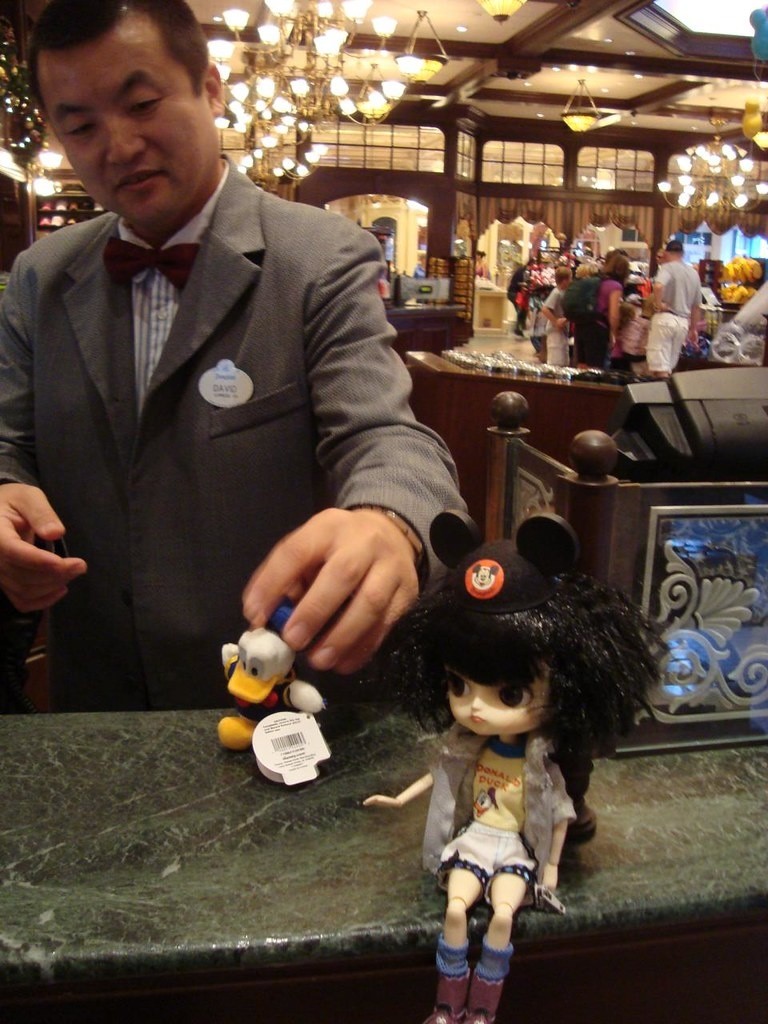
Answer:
[[562, 276, 613, 326]]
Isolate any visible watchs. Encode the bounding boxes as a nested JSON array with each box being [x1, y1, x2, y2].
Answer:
[[348, 506, 425, 570]]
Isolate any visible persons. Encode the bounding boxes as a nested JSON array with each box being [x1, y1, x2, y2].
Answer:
[[507, 259, 535, 337], [541, 241, 702, 379], [0, 0, 469, 716], [363, 514, 659, 1023], [413, 250, 426, 278], [475, 250, 492, 282]]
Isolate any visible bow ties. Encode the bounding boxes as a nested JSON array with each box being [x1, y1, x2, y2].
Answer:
[[103, 236, 201, 292]]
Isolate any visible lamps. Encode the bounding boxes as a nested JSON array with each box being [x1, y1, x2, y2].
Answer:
[[478, 0, 528, 21], [395, 10, 448, 83], [558, 79, 603, 132], [658, 117, 768, 215], [207, 0, 425, 182]]
